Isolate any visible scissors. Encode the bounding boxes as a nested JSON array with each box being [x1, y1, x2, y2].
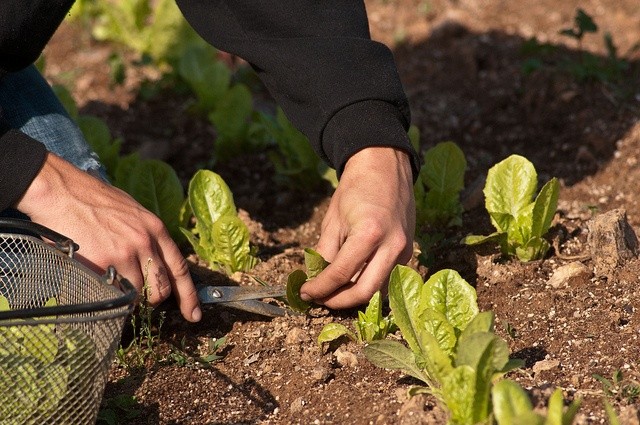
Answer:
[[153, 281, 302, 317]]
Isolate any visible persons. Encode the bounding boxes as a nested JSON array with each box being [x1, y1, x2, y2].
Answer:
[[0, 2, 421, 322]]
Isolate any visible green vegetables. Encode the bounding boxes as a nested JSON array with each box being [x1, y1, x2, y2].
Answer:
[[0, 293, 99, 425]]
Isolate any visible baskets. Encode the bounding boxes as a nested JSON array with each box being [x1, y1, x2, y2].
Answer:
[[0, 217, 138, 425]]
